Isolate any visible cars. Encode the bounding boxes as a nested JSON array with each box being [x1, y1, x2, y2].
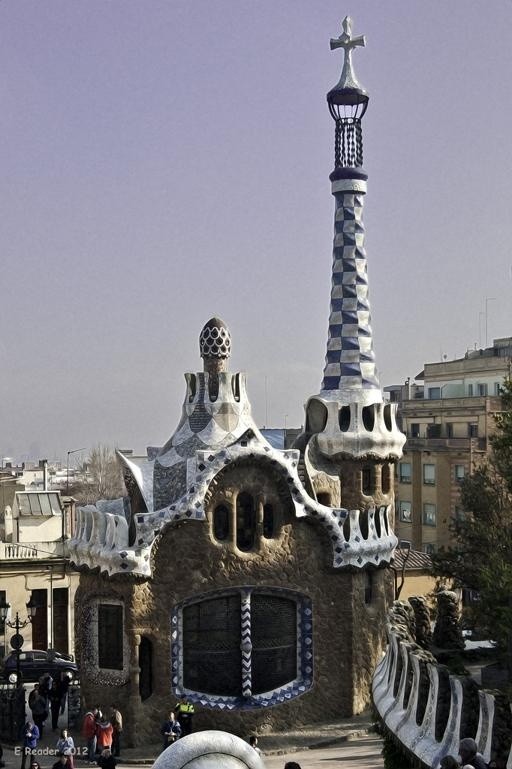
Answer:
[[1, 651, 80, 684]]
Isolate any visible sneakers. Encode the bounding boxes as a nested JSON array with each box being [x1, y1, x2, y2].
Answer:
[[89, 761, 95, 764], [84, 761, 88, 764]]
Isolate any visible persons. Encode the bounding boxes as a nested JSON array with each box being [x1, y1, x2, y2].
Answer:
[[285, 762, 301, 769], [435, 737, 500, 769], [20, 673, 121, 769], [249, 736, 261, 756], [174, 693, 194, 739], [160, 711, 181, 752]]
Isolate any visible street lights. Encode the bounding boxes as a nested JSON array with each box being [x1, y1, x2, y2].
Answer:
[[6, 612, 31, 684]]
